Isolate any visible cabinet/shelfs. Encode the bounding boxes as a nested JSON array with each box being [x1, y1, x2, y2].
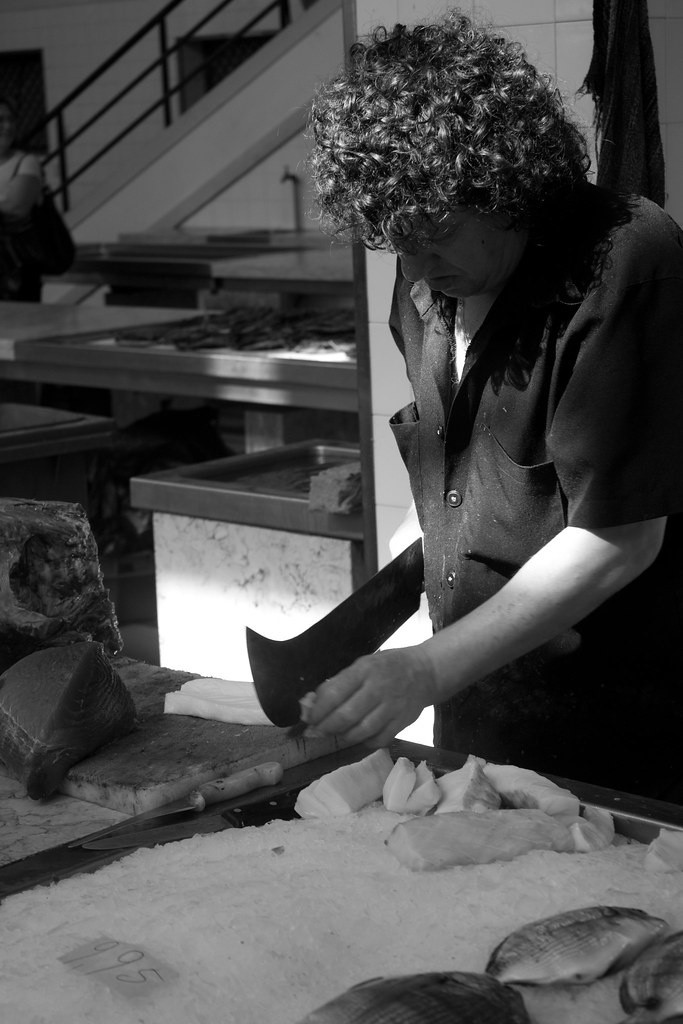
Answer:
[[130, 436, 368, 729], [0, 403, 118, 522]]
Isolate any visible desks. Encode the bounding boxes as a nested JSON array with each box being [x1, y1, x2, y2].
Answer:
[[76, 246, 355, 308], [0, 300, 359, 454]]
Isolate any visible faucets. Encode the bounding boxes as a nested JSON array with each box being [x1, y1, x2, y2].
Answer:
[[277, 164, 303, 232]]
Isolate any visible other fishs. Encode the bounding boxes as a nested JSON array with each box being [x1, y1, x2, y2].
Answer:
[[478, 907, 669, 988], [620, 930, 682, 1024]]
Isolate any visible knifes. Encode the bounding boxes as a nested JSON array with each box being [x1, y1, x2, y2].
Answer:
[[82, 787, 298, 850], [246, 535, 426, 728], [67, 760, 285, 848]]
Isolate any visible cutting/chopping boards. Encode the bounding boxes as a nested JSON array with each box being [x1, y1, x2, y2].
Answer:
[[60, 653, 361, 818]]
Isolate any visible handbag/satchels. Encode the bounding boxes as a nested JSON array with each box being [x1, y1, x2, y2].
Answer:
[[4, 154, 75, 276]]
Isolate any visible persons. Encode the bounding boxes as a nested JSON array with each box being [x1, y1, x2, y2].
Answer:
[[304, 6, 683, 828], [1, 96, 47, 302]]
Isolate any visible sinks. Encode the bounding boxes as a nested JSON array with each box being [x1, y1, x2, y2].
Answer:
[[176, 441, 360, 500]]
